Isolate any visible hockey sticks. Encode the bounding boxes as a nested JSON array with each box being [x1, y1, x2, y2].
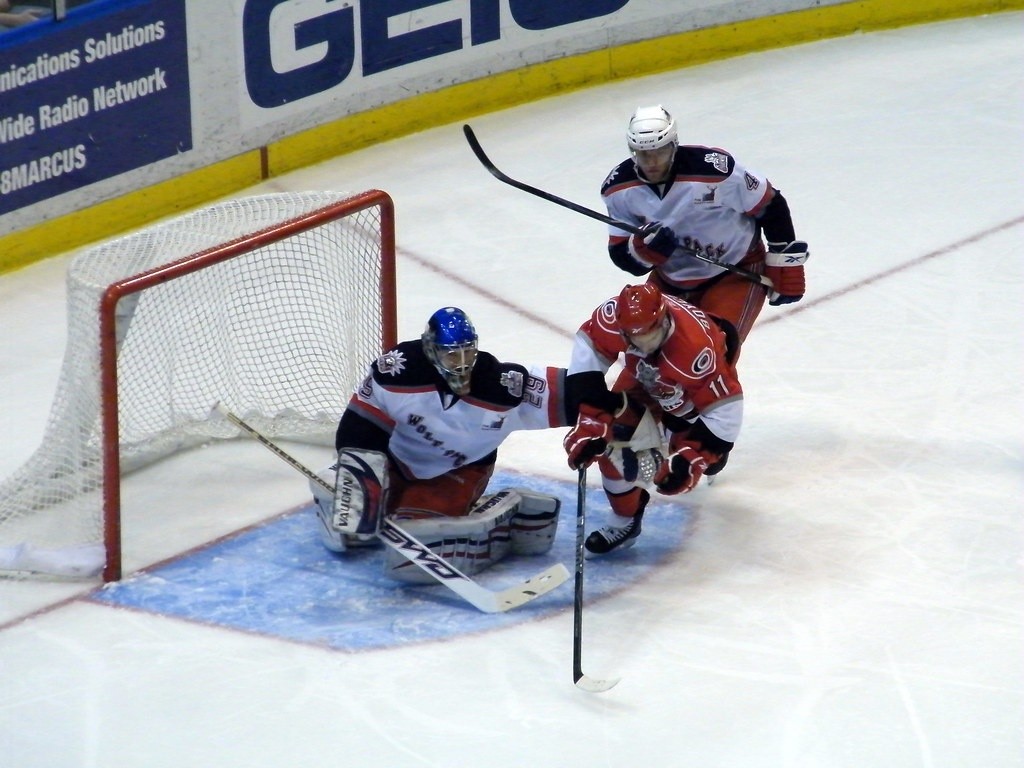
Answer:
[[211, 397, 573, 616], [460, 122, 776, 290], [570, 464, 624, 695]]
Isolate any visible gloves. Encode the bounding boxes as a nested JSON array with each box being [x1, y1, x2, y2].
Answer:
[[563, 403, 616, 470], [765, 241, 809, 306], [653, 439, 723, 496], [609, 220, 678, 277]]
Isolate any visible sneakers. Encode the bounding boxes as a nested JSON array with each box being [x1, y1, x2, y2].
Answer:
[[584, 489, 650, 559]]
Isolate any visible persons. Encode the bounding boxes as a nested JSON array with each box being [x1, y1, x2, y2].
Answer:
[[334, 308, 567, 538], [562, 284, 745, 559], [599, 105, 810, 344]]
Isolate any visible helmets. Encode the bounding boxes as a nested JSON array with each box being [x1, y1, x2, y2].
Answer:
[[422, 306, 478, 395], [626, 104, 679, 167], [615, 283, 668, 337]]
